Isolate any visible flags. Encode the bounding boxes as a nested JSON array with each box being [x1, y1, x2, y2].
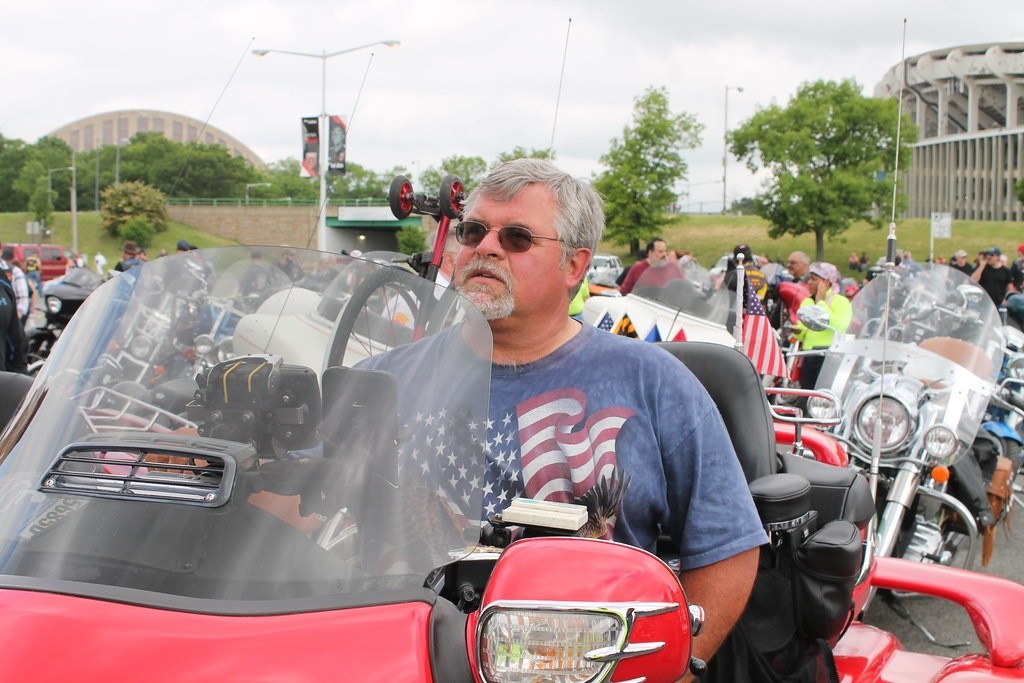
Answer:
[[741, 272, 789, 378]]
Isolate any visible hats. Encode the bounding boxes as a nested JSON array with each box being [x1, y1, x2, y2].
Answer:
[[120, 241, 141, 254], [986, 247, 1002, 257], [735, 245, 750, 255], [809, 262, 837, 282], [1017, 244, 1024, 251]]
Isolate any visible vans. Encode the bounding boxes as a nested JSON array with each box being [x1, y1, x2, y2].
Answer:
[[0, 242, 77, 283], [586, 255, 625, 288]]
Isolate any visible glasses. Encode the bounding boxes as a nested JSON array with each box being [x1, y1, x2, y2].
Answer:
[[456, 220, 590, 253]]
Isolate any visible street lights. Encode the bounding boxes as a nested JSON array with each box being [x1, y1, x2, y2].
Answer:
[[250, 38, 402, 254], [47, 167, 75, 212], [721, 83, 750, 214], [93, 139, 131, 215]]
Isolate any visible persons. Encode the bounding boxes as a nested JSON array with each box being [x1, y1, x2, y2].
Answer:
[[237, 244, 361, 294], [0, 247, 41, 330], [150, 158, 771, 682], [381, 224, 461, 330], [849, 245, 1024, 310], [617, 235, 852, 390], [66, 252, 106, 275], [115, 240, 215, 294]]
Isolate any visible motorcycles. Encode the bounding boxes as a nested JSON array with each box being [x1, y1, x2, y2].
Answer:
[[0, 238, 1024, 683]]
